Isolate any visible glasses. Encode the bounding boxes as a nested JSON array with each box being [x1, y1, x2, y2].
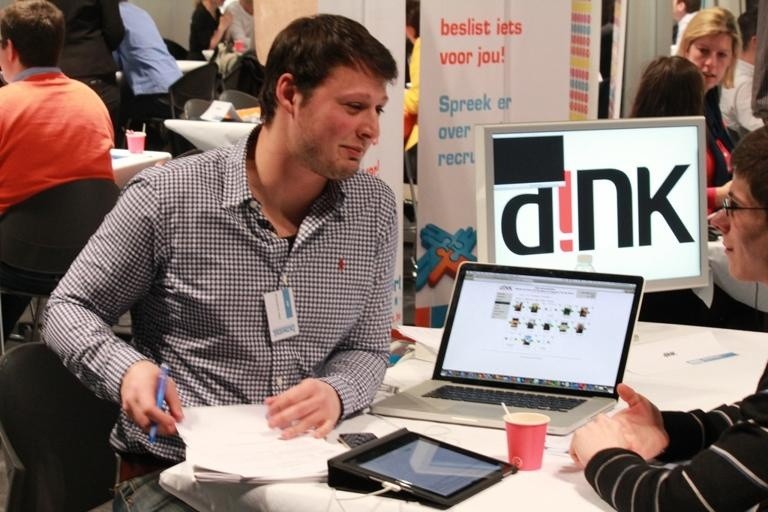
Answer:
[[721, 199, 767, 216]]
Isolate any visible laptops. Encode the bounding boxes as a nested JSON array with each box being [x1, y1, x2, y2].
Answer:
[[368, 260, 647, 436]]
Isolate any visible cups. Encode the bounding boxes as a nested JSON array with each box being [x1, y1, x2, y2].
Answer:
[[125, 131, 146, 155], [502, 412, 551, 470]]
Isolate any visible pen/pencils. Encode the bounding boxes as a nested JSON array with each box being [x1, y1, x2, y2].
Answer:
[[149, 362, 170, 443]]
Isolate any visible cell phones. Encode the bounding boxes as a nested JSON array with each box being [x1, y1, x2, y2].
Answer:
[[339, 432, 378, 449]]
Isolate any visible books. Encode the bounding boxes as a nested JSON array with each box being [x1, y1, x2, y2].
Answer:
[[175, 403, 351, 486]]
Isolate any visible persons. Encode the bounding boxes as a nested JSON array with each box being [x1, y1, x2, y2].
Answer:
[[0, 0, 114, 214], [40, 12, 400, 511], [406, 2, 417, 227], [567, 127, 768, 512], [631, 0, 767, 237], [52, 0, 258, 152]]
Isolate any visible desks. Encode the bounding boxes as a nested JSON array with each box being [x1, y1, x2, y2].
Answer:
[[159, 321, 768, 512]]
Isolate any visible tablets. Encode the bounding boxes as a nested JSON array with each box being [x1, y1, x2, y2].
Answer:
[[326, 427, 520, 509]]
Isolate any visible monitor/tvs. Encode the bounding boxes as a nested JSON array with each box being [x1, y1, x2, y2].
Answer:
[[475, 115, 712, 296]]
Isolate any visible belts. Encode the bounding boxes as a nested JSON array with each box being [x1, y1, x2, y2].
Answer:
[[112, 446, 176, 485]]
